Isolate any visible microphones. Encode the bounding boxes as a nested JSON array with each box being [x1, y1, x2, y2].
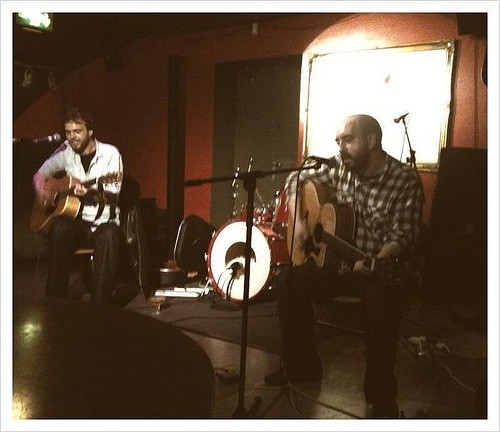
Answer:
[[311, 156, 339, 168], [32, 133, 61, 144], [395, 114, 407, 123]]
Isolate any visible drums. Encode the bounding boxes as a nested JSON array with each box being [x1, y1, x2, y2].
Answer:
[[238, 202, 275, 223], [272, 185, 289, 237], [204, 218, 293, 305]]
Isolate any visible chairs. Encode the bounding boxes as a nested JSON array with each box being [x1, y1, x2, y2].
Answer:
[[312, 222, 433, 329]]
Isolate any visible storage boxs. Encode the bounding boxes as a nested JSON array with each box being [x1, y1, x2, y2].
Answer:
[[426, 147, 488, 304]]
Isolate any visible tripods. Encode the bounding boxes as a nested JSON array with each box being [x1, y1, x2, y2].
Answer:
[[184, 162, 325, 419]]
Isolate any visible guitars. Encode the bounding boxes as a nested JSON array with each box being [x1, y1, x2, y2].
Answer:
[[29, 171, 122, 234], [285, 177, 416, 291]]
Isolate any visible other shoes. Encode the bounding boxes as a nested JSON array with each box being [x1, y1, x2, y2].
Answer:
[[52, 282, 69, 298], [371, 389, 399, 418], [264, 362, 323, 385]]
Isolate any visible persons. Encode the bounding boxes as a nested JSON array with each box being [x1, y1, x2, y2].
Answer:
[[264, 115, 424, 419], [35, 108, 125, 312]]
